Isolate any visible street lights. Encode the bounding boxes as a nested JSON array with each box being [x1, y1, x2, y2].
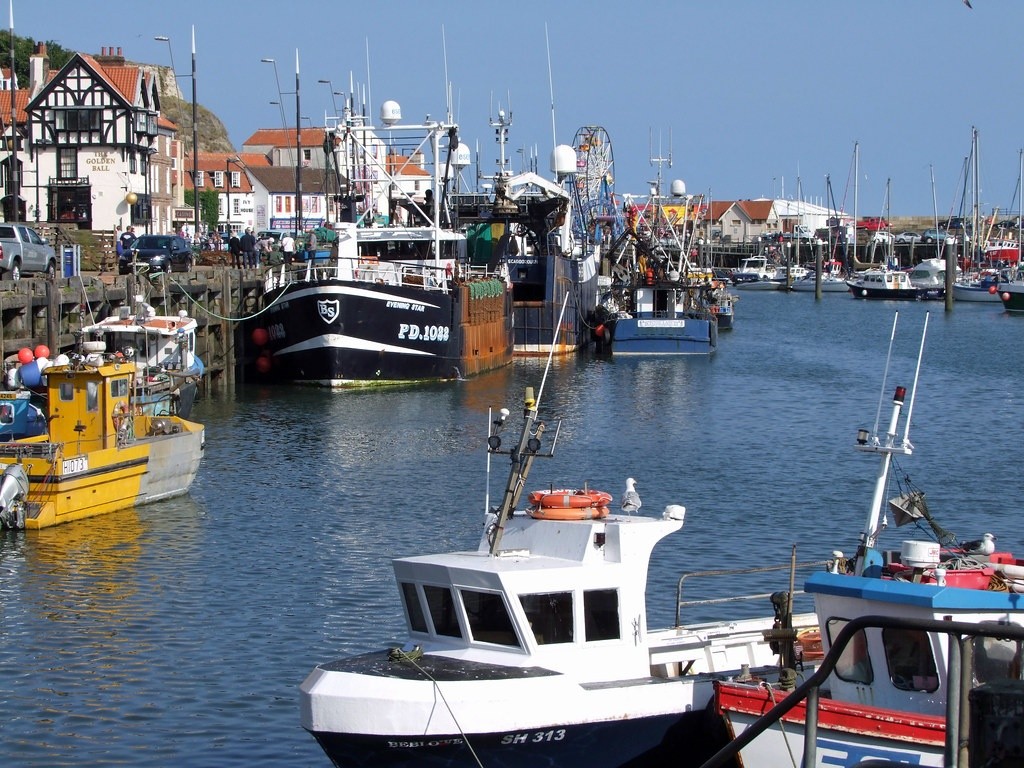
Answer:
[[260, 47, 303, 232], [302, 70, 368, 220], [144, 147, 160, 234], [153, 24, 199, 237], [226, 157, 240, 250]]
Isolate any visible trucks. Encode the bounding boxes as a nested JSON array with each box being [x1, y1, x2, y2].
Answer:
[[761, 225, 817, 243]]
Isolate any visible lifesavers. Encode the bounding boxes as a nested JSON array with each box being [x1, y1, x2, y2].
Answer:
[[528, 489, 612, 507], [355, 268, 359, 279], [446, 263, 452, 276], [113, 401, 129, 431], [529, 505, 609, 520]]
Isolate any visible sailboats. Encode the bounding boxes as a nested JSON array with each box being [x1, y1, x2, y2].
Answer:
[[738, 127, 1024, 314]]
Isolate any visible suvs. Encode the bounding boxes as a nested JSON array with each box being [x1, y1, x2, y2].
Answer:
[[856, 217, 886, 231], [935, 217, 971, 229], [871, 231, 895, 243], [922, 229, 955, 243], [0, 224, 57, 280]]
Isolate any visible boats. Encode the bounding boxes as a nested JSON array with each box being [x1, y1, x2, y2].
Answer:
[[0, 249, 204, 441], [0, 361, 204, 530], [711, 307, 1024, 768], [300, 292, 998, 768], [271, 20, 738, 391]]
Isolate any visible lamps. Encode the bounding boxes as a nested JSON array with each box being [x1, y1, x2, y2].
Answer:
[[122, 186, 137, 205]]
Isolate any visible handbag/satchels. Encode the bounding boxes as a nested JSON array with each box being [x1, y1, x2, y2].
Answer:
[[280, 246, 284, 252]]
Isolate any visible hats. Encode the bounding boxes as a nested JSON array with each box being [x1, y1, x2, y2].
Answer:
[[268, 237, 275, 243]]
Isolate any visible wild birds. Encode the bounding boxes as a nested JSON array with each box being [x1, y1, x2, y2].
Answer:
[[962, 0, 974, 9], [962, 532, 998, 556], [621, 478, 642, 516]]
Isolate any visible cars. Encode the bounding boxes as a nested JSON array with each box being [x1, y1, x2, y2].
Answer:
[[993, 221, 1015, 229], [118, 235, 193, 274], [895, 232, 922, 244]]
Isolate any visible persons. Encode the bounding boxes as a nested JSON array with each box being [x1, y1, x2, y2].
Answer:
[[116, 226, 137, 251], [176, 228, 318, 269], [72, 206, 87, 220]]
